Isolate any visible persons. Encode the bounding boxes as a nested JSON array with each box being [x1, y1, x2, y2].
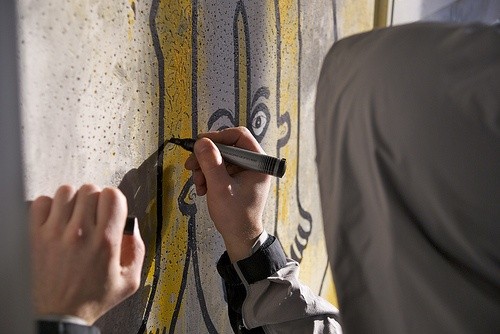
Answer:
[[25, 17, 499, 333]]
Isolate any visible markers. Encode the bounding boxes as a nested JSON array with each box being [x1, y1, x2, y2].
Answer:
[[170, 136, 288, 179]]
